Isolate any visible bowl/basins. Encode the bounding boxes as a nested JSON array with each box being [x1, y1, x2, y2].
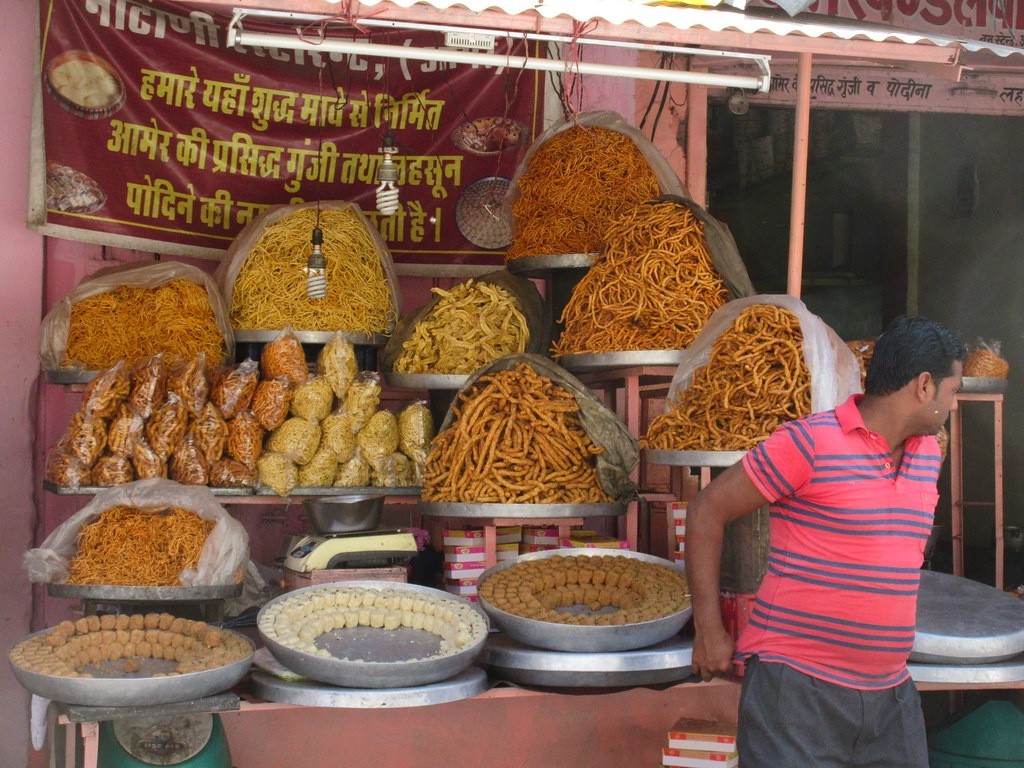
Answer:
[[301, 494, 386, 534]]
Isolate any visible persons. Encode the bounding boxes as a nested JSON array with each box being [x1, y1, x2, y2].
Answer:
[[686, 318, 967, 768]]
